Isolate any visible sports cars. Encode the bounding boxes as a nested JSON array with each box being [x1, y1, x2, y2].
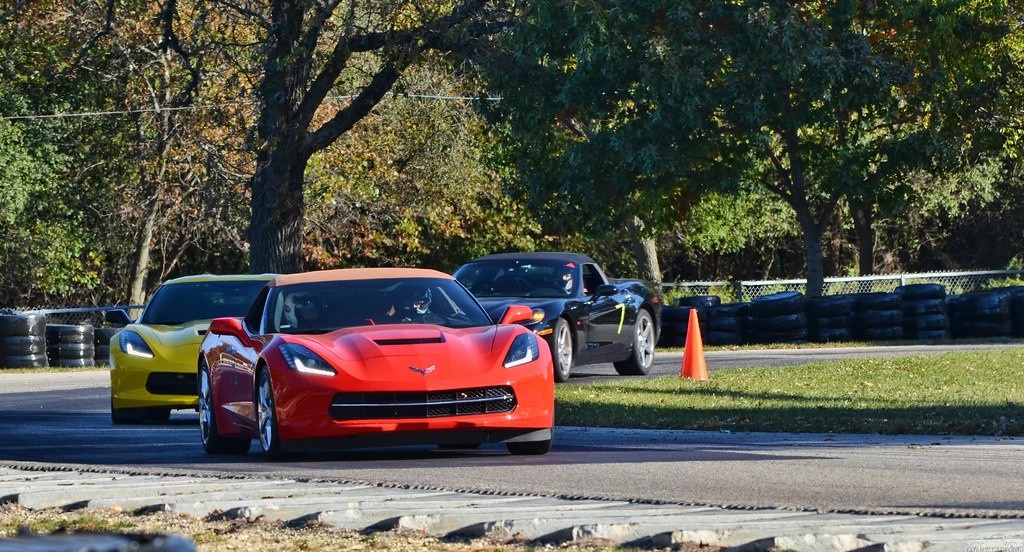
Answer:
[[452, 250, 663, 382], [103, 273, 278, 424], [196, 268, 554, 456]]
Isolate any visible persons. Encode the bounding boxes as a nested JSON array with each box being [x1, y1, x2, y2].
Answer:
[[545, 262, 588, 297], [379, 283, 439, 324], [284, 289, 327, 332]]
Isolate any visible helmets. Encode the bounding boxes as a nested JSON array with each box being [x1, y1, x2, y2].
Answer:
[[557, 263, 576, 287], [408, 287, 432, 315], [284, 290, 328, 329]]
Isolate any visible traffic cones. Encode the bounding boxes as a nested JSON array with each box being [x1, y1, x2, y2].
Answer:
[[678, 308, 710, 381]]
